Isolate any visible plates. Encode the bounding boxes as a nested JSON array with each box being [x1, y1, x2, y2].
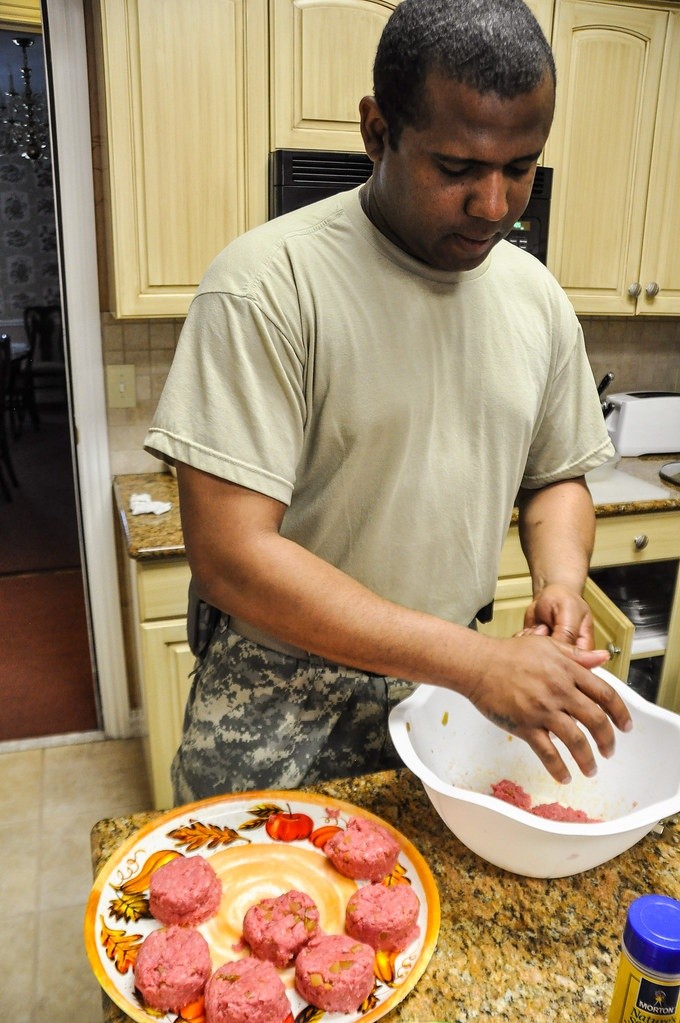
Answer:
[[83, 791, 441, 1023]]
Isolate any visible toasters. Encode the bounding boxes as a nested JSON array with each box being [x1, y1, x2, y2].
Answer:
[[603, 389, 680, 458]]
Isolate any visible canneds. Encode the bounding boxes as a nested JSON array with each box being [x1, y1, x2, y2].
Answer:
[[608, 895, 680, 1023]]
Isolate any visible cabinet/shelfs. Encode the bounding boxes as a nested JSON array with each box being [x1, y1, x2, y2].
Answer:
[[120, 510, 680, 811], [85, 0, 680, 322]]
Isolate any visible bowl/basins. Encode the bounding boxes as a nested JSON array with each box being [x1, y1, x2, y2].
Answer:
[[389, 663, 679, 879]]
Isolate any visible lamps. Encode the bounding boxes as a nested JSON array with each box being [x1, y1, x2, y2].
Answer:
[[0, 38, 51, 162]]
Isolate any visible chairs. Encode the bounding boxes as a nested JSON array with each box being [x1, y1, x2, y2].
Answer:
[[0, 333, 21, 507], [9, 305, 77, 440]]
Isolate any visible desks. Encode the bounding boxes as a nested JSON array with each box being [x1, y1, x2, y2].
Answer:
[[9, 340, 32, 434], [88, 768, 680, 1023]]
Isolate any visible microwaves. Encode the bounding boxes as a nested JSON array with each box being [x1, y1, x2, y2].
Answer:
[[266, 149, 553, 273]]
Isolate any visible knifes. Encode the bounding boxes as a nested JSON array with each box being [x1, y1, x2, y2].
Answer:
[[597, 371, 615, 420]]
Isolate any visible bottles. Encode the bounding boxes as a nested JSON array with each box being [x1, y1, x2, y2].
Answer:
[[608, 894, 679, 1023]]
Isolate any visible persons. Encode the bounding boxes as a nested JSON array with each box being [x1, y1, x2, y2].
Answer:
[[145, 0, 634, 807]]
